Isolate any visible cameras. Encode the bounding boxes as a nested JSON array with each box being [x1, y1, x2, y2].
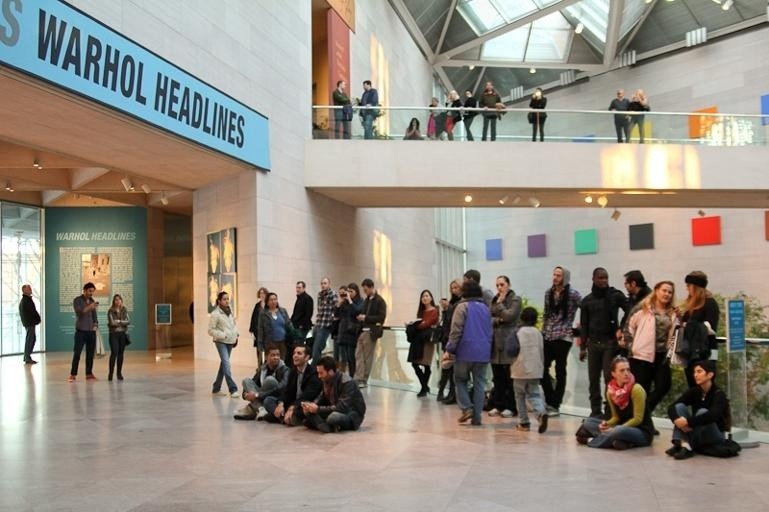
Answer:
[[341, 294, 347, 297]]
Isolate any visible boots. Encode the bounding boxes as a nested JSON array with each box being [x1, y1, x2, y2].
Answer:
[[415, 369, 431, 397]]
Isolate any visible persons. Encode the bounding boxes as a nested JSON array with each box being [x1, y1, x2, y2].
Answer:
[[665, 272, 720, 413], [69, 283, 99, 381], [578, 269, 627, 419], [408, 290, 438, 396], [360, 80, 377, 137], [19, 286, 40, 364], [627, 88, 650, 143], [540, 268, 583, 417], [440, 270, 549, 434], [403, 117, 421, 139], [208, 292, 240, 399], [334, 80, 350, 138], [622, 281, 676, 433], [234, 279, 386, 433], [616, 270, 653, 353], [426, 81, 504, 140], [666, 359, 741, 458], [530, 87, 547, 141], [106, 294, 131, 382], [575, 356, 661, 449], [609, 90, 629, 142]]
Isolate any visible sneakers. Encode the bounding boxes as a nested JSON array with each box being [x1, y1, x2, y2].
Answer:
[[488, 406, 513, 417], [68, 373, 126, 384], [212, 390, 240, 398], [515, 423, 531, 432], [537, 414, 549, 434], [436, 384, 457, 404]]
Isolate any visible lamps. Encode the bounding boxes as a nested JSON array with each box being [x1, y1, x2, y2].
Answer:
[[722, 0, 734, 11], [463, 191, 621, 222], [5, 183, 15, 193], [119, 174, 169, 206], [575, 24, 584, 34], [529, 66, 536, 73], [33, 159, 43, 171]]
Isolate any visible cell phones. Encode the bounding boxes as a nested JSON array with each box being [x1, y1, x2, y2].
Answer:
[[95, 301, 99, 305]]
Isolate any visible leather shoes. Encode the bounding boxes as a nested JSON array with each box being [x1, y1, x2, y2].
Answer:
[[457, 409, 482, 426], [674, 447, 695, 460], [576, 435, 591, 444], [666, 445, 679, 456], [23, 357, 37, 364], [613, 439, 633, 450], [233, 403, 340, 432]]
